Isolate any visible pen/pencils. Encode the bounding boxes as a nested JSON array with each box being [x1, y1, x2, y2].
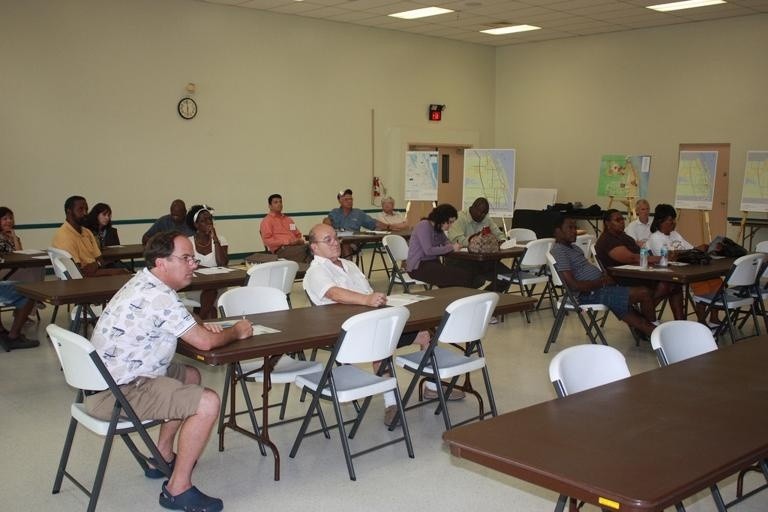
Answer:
[[456, 239, 459, 243], [208, 267, 222, 270], [241, 312, 246, 320]]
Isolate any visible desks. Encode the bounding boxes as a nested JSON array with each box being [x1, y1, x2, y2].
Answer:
[[442, 335, 767, 511], [303, 228, 413, 269], [446, 247, 524, 291], [176, 286, 538, 481], [610, 251, 768, 323], [0, 244, 145, 280], [16, 261, 311, 335]]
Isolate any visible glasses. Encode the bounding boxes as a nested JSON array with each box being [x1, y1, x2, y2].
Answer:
[[171, 254, 201, 266], [311, 237, 340, 245]]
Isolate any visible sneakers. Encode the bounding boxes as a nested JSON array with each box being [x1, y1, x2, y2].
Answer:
[[424, 386, 465, 400], [384, 401, 400, 425], [3, 337, 39, 350]]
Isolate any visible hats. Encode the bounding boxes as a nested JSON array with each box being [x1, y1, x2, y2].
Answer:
[[338, 188, 353, 198]]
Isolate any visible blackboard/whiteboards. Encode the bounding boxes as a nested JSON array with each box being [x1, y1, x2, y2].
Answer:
[[515, 188, 556, 210]]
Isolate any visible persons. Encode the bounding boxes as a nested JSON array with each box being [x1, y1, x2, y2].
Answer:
[[323, 187, 396, 261], [624, 198, 654, 249], [0, 206, 47, 327], [183, 205, 229, 320], [82, 231, 254, 512], [141, 199, 194, 246], [548, 213, 665, 339], [447, 196, 514, 325], [82, 202, 121, 247], [50, 195, 130, 278], [259, 194, 312, 262], [301, 222, 466, 427], [0, 280, 41, 352], [376, 196, 409, 231], [593, 209, 685, 321], [645, 203, 722, 327], [405, 202, 474, 288]]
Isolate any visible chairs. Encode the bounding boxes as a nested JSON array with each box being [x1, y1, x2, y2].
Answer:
[[389, 292, 498, 429], [218, 288, 330, 452], [289, 308, 415, 481], [725, 241, 768, 334], [47, 259, 97, 338], [507, 228, 559, 308], [536, 235, 596, 315], [45, 324, 174, 511], [651, 321, 767, 512], [300, 281, 362, 413], [496, 239, 558, 323], [548, 345, 686, 511], [701, 254, 765, 345], [543, 251, 608, 352], [382, 235, 429, 295], [245, 261, 298, 308]]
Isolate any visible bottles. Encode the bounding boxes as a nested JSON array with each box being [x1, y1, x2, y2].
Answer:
[[639, 244, 648, 268], [660, 243, 668, 266]]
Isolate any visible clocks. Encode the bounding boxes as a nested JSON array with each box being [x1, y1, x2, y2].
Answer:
[[178, 98, 198, 119]]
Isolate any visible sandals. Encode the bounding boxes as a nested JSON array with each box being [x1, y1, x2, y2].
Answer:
[[159, 480, 223, 512], [145, 454, 176, 478]]
